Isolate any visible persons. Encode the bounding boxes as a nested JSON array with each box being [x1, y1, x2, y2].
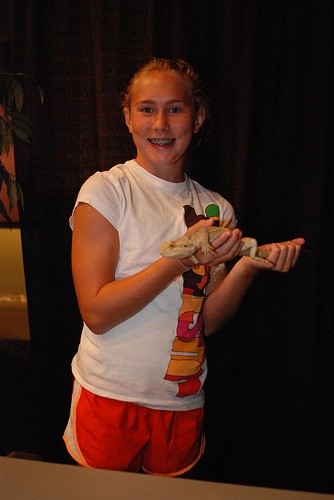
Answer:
[[62, 60, 306, 479]]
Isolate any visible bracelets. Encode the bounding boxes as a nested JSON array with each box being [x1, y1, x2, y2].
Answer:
[[176, 259, 191, 271], [190, 254, 199, 264]]
